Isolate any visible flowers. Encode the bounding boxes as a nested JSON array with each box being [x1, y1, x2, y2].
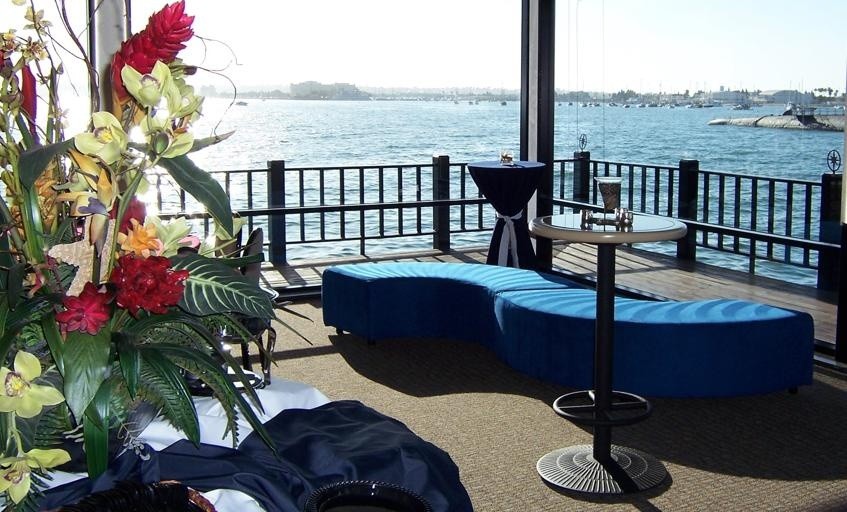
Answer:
[[0, 0, 316, 508]]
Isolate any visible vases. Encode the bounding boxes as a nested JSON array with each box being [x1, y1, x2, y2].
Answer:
[[4, 357, 181, 473]]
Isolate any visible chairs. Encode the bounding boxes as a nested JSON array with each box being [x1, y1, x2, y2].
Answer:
[[221, 227, 267, 372], [215, 210, 241, 268]]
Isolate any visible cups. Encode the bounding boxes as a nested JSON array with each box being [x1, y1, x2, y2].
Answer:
[[500, 150, 514, 165], [580, 209, 594, 223], [621, 213, 633, 226], [615, 207, 627, 219]]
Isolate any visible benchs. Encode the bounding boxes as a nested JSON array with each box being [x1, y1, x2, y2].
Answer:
[[322, 263, 814, 400]]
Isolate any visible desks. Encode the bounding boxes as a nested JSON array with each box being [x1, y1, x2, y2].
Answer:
[[467, 163, 546, 270], [0, 368, 334, 511], [529, 214, 688, 498]]
